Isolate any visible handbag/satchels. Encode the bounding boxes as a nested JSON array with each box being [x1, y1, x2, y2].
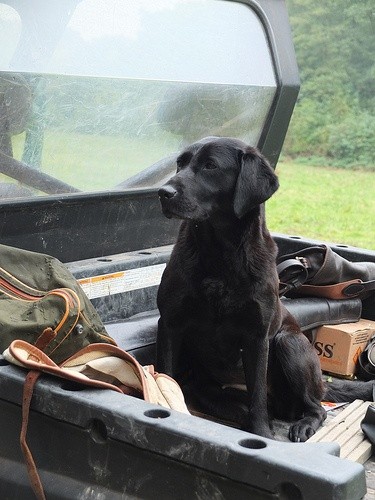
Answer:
[[278, 244, 375, 300], [0, 244, 117, 368], [3, 336, 192, 417]]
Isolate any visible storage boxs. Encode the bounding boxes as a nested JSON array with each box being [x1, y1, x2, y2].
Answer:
[[312, 318, 375, 375]]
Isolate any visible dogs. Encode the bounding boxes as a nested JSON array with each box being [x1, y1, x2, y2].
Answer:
[[156, 136, 375, 442]]
